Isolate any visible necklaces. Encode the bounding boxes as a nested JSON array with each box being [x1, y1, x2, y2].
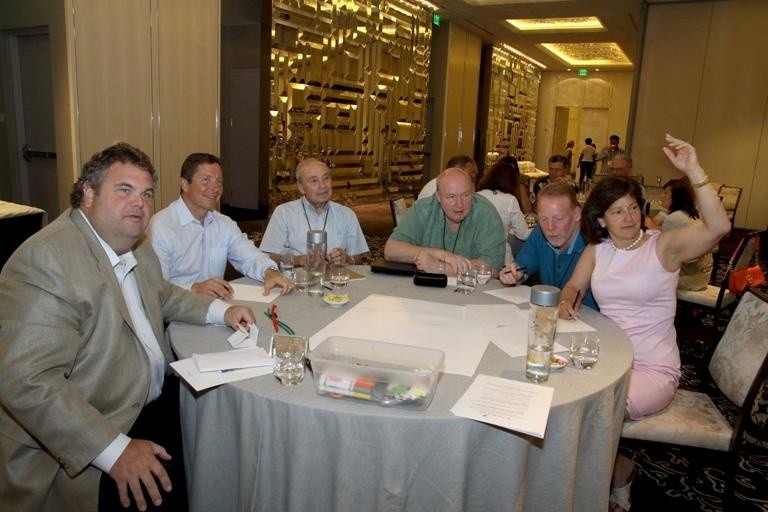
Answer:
[[609, 227, 643, 253]]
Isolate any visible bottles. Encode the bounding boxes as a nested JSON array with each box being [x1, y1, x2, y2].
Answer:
[[278, 241, 297, 284], [306, 229, 325, 297], [524, 285, 562, 382]]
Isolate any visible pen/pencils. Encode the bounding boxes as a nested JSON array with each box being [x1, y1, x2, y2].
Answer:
[[504, 267, 526, 274], [568, 289, 581, 320], [320, 371, 375, 400]]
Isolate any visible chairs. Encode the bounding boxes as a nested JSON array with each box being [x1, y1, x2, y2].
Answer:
[[389, 195, 408, 226]]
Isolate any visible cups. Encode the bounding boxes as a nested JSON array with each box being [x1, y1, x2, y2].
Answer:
[[272, 346, 307, 385], [330, 262, 352, 288], [611, 144, 617, 152], [569, 333, 600, 371], [576, 190, 590, 202], [458, 263, 491, 293]]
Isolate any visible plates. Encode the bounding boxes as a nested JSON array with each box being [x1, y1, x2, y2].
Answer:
[[523, 214, 538, 229]]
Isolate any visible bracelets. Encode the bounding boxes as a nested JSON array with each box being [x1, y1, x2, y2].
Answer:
[[690, 175, 709, 188], [439, 262, 445, 275]]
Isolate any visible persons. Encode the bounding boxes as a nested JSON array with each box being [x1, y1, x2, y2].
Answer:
[[256, 157, 370, 268], [383, 167, 507, 282], [655, 172, 711, 290], [499, 182, 600, 314], [0, 143, 259, 511], [448, 133, 650, 214], [145, 153, 297, 299], [548, 132, 735, 511]]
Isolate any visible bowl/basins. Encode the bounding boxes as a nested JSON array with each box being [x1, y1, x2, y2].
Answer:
[[322, 297, 349, 309], [551, 355, 568, 371]]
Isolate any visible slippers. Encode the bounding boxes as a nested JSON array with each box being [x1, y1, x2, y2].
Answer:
[[608, 463, 637, 511]]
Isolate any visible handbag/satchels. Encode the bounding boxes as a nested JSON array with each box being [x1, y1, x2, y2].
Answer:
[[729, 266, 765, 293]]
